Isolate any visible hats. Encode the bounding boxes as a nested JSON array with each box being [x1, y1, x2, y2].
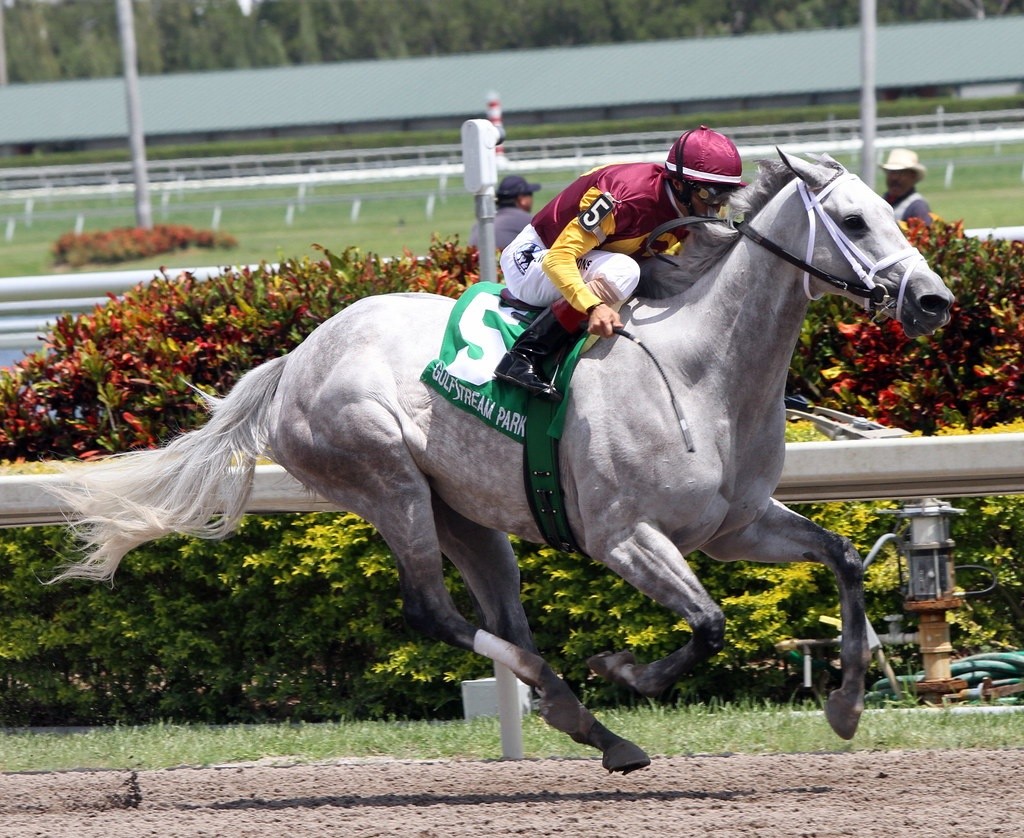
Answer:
[[663, 125, 749, 187], [495, 175, 542, 196], [878, 148, 926, 183]]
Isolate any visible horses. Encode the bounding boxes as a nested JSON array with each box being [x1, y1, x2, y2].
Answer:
[[30, 142, 957, 773]]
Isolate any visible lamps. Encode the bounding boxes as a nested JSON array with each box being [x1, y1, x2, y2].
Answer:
[[899, 496, 966, 603]]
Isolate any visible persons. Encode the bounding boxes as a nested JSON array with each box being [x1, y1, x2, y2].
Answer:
[[493, 124, 749, 404], [877, 147, 933, 227], [467, 175, 541, 250]]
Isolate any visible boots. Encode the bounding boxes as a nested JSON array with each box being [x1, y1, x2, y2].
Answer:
[[494, 307, 569, 403]]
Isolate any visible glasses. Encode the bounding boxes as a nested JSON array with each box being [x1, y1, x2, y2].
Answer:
[[671, 173, 731, 208]]
